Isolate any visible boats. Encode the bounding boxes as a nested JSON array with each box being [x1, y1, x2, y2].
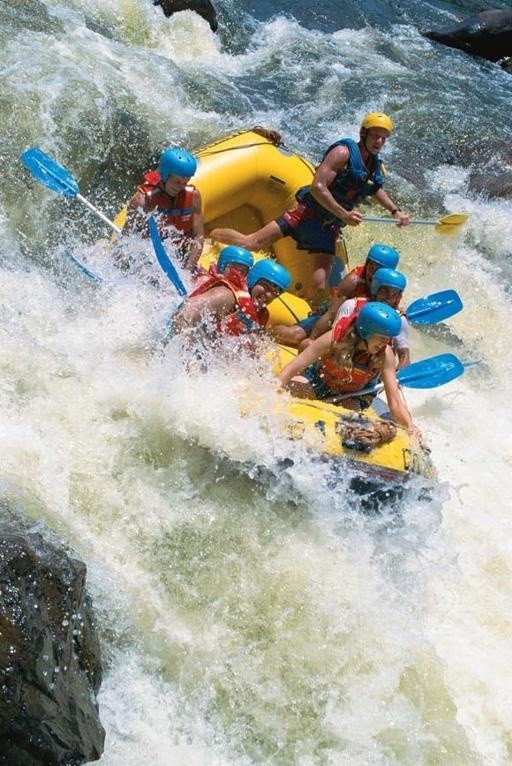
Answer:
[[106, 128, 439, 501]]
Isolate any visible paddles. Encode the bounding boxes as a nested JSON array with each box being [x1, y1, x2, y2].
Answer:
[[323, 353, 464, 403], [405, 290, 463, 326], [361, 213, 469, 235], [19, 146, 123, 236]]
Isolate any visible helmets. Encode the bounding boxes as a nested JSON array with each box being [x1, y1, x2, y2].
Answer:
[[218, 246, 255, 272], [159, 147, 197, 183], [247, 259, 291, 291], [356, 301, 401, 341], [370, 267, 406, 294], [361, 113, 393, 138], [368, 243, 399, 269]]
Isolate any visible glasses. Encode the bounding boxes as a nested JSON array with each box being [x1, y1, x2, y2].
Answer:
[[259, 282, 280, 298]]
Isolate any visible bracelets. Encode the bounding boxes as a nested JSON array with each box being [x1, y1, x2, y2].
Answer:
[[393, 208, 401, 214]]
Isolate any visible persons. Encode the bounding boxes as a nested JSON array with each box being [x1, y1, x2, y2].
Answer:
[[276, 302, 417, 433], [210, 111, 410, 291], [122, 147, 204, 272], [217, 245, 254, 277], [272, 243, 399, 347], [299, 268, 409, 371], [174, 259, 292, 371]]
